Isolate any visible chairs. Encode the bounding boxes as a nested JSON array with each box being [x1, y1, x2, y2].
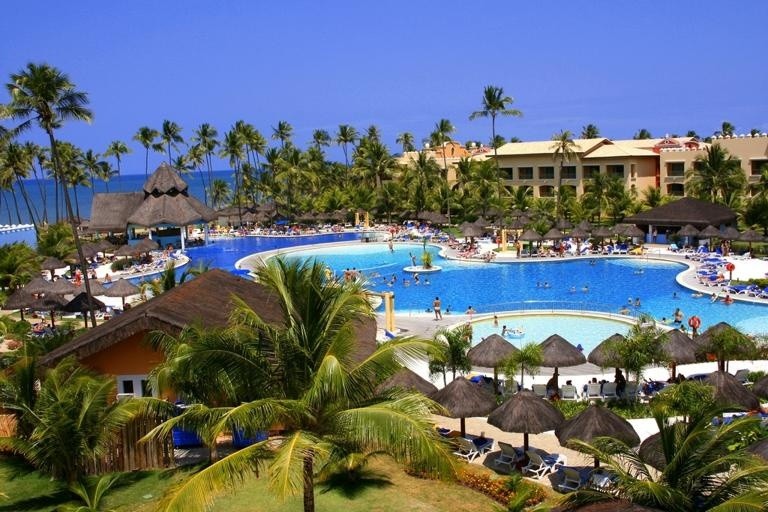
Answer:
[[385, 329, 409, 337]]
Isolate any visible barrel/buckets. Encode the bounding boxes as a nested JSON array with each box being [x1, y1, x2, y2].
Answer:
[[231, 424, 269, 450], [171, 401, 206, 449]]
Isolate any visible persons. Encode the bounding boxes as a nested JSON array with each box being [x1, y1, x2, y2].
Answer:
[[653, 228, 657, 243], [572, 286, 577, 293], [544, 281, 549, 288], [68, 231, 203, 286], [583, 286, 589, 293], [325, 266, 430, 288], [665, 229, 670, 243], [623, 296, 667, 323], [208, 224, 521, 258], [671, 240, 768, 331], [536, 282, 542, 287], [424, 296, 512, 339]]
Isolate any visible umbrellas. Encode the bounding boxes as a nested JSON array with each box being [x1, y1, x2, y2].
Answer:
[[486, 389, 562, 457], [675, 224, 765, 250], [0, 276, 142, 330], [468, 323, 745, 394], [375, 365, 437, 402], [427, 376, 496, 440], [558, 401, 640, 468]]
